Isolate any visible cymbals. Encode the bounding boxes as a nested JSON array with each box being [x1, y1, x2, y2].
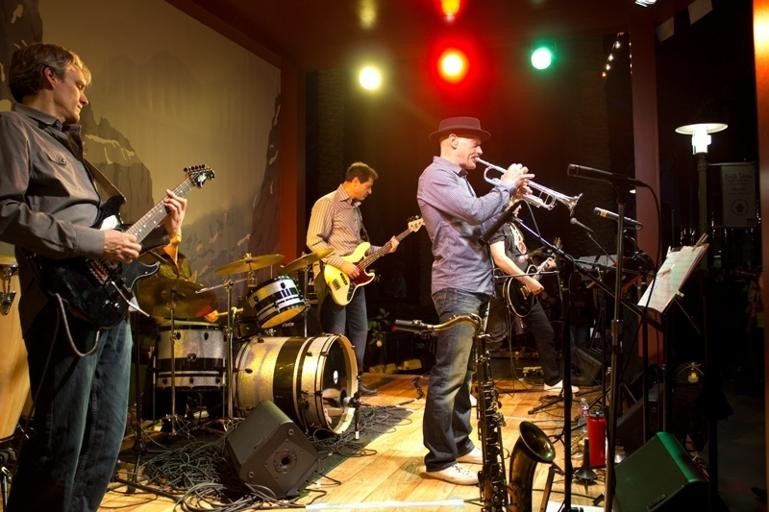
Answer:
[[284, 248, 334, 272], [138, 279, 219, 319], [214, 254, 285, 276]]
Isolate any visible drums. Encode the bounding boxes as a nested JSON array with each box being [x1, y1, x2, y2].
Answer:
[[234, 275, 306, 340], [232, 334, 359, 435], [153, 324, 228, 392]]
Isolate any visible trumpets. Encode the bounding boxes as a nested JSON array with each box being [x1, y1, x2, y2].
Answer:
[[474, 156, 583, 217]]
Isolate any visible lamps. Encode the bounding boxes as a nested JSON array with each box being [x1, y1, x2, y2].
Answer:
[[440, 0, 460, 24], [440, 52, 467, 81], [356, 62, 382, 90], [531, 45, 552, 71], [675, 122, 727, 238]]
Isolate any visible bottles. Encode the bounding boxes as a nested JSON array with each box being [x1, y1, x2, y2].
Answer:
[[577, 397, 590, 434]]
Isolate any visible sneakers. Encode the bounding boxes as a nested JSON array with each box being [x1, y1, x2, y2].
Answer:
[[425, 463, 480, 485], [544, 379, 580, 393], [468, 392, 478, 407], [455, 446, 502, 464]]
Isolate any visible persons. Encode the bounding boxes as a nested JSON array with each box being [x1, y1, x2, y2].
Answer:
[[306, 162, 400, 396], [486, 203, 581, 394], [416, 116, 536, 486], [0, 42, 188, 512]]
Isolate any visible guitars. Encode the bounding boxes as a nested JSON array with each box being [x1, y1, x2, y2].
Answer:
[[24, 165, 215, 329], [323, 215, 426, 306], [495, 236, 563, 318]]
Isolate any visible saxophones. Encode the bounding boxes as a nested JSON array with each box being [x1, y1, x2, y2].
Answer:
[[391, 312, 555, 512]]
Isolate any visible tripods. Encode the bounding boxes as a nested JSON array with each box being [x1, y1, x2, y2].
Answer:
[[527, 270, 638, 424], [118, 280, 240, 495]]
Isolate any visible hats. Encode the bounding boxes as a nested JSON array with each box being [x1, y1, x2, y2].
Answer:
[[428, 116, 493, 143]]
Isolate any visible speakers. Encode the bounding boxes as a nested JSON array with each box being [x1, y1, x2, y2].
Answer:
[[225, 397, 320, 501], [612, 431, 710, 511]]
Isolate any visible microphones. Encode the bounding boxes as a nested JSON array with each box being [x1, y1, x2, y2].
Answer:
[[479, 202, 524, 247], [593, 206, 637, 227], [569, 218, 589, 231], [567, 165, 645, 190]]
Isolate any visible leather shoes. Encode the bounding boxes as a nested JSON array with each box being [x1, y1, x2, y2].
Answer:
[[358, 381, 378, 396]]
